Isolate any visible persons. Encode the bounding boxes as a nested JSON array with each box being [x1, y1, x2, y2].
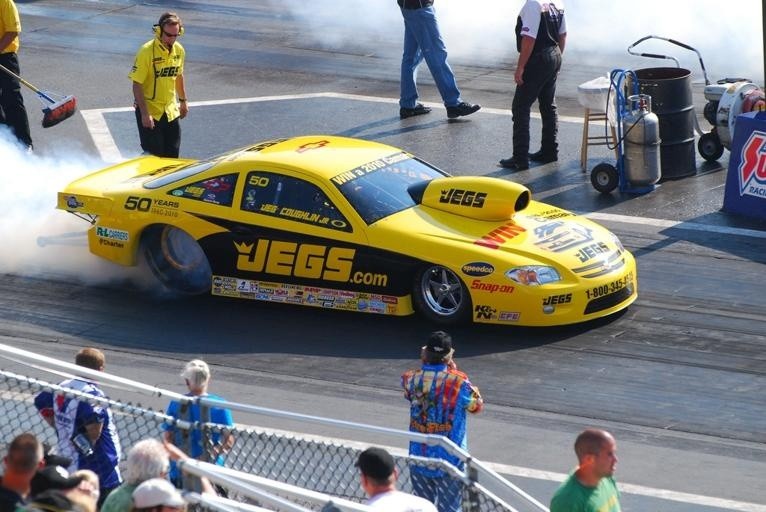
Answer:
[[159, 359, 235, 490], [397, 0, 482, 120], [353, 447, 440, 512], [33, 347, 124, 512], [0, 0, 34, 156], [127, 10, 189, 159], [549, 429, 623, 512], [399, 330, 484, 512], [0, 431, 188, 512], [500, 0, 568, 171]]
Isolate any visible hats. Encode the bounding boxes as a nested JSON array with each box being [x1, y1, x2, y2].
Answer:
[[132, 479, 184, 508], [31, 467, 84, 493], [354, 448, 394, 477], [422, 331, 451, 353]]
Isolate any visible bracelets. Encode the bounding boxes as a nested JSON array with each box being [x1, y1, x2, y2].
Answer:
[[179, 97, 187, 102]]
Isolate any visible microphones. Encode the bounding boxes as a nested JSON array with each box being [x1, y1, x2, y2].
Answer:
[[161, 38, 172, 48]]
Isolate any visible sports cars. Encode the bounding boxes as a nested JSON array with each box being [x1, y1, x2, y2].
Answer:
[[54, 134, 639, 329]]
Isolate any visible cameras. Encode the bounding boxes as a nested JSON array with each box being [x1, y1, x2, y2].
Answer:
[[70, 432, 92, 457]]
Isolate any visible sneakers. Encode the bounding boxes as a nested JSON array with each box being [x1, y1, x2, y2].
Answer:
[[500, 157, 528, 170], [446, 102, 480, 118], [401, 103, 431, 117], [528, 149, 557, 162]]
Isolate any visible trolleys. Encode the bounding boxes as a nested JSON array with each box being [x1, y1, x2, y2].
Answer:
[[590, 68, 656, 194], [626, 34, 765, 162]]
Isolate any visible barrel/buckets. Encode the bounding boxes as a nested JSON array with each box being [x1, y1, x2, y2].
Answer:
[[627, 66, 698, 180]]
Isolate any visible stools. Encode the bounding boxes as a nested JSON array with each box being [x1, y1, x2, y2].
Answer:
[[578, 83, 618, 172]]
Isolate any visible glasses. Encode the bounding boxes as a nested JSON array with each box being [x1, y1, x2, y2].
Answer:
[[161, 30, 179, 37]]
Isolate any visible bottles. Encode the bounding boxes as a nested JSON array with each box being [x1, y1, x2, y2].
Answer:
[[72, 433, 93, 457]]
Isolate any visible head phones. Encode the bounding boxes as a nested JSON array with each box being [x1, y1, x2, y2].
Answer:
[[151, 16, 185, 38]]
